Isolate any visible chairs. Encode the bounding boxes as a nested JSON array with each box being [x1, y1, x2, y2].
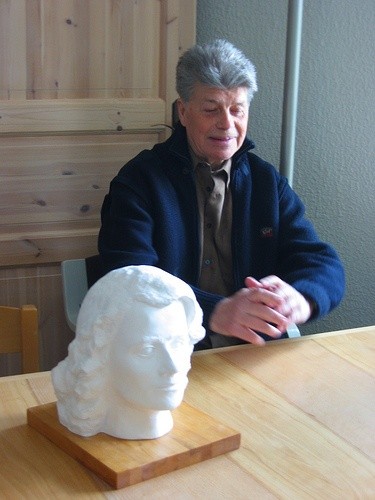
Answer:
[[1, 302, 42, 377], [61, 254, 105, 331]]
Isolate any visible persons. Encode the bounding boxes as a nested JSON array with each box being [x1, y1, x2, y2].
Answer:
[[51, 264, 206, 441], [97, 37, 345, 353]]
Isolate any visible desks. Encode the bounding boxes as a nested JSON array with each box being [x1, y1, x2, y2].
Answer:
[[1, 324, 373, 500]]
